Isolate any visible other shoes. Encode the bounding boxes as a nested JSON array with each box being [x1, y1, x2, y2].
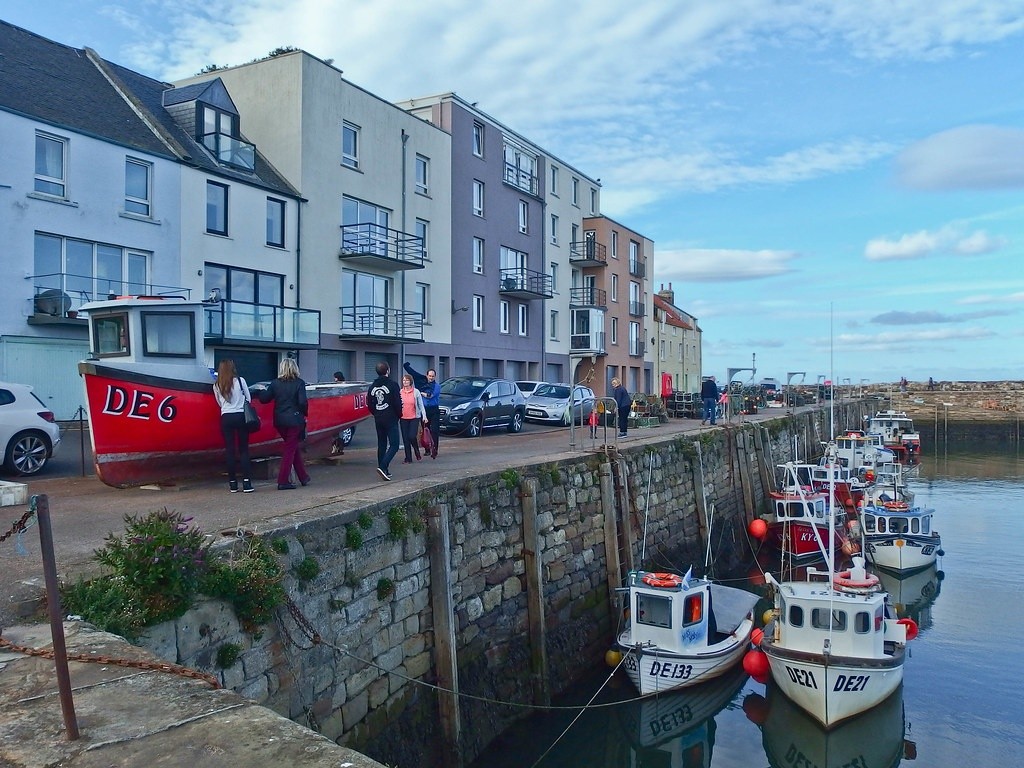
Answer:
[[376, 467, 391, 481], [302, 477, 311, 486], [424, 450, 431, 456], [229, 482, 239, 492], [700, 421, 705, 425], [402, 459, 412, 464], [616, 432, 628, 439], [589, 435, 598, 439], [243, 481, 255, 493], [417, 456, 423, 461], [278, 483, 298, 490], [386, 470, 392, 477], [710, 422, 717, 426], [431, 450, 438, 459]]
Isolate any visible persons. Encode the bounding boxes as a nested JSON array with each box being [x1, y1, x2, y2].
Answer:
[[404, 362, 441, 460], [928, 377, 934, 391], [334, 372, 345, 382], [768, 389, 773, 394], [718, 391, 729, 418], [399, 374, 428, 465], [213, 358, 255, 493], [368, 361, 403, 481], [900, 377, 908, 392], [589, 407, 599, 440], [611, 377, 632, 439], [777, 389, 780, 394], [701, 376, 719, 425], [257, 357, 310, 490]]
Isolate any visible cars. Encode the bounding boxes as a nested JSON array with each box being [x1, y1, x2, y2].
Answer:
[[437, 375, 527, 438], [525, 383, 596, 427], [514, 380, 550, 399], [0, 382, 61, 477]]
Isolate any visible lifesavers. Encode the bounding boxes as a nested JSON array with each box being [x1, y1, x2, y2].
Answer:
[[887, 507, 909, 512], [882, 501, 908, 508], [833, 570, 879, 587], [833, 582, 882, 595], [642, 572, 683, 588]]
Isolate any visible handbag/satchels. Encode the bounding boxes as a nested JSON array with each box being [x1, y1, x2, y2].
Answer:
[[420, 421, 434, 448], [237, 376, 260, 432], [300, 419, 306, 441]]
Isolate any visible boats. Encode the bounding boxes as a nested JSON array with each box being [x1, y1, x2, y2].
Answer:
[[858, 463, 942, 576], [805, 408, 921, 523], [613, 450, 763, 696], [763, 434, 852, 561], [615, 659, 752, 754], [864, 561, 945, 617], [78, 358, 375, 489], [758, 298, 908, 731], [761, 676, 906, 768]]
